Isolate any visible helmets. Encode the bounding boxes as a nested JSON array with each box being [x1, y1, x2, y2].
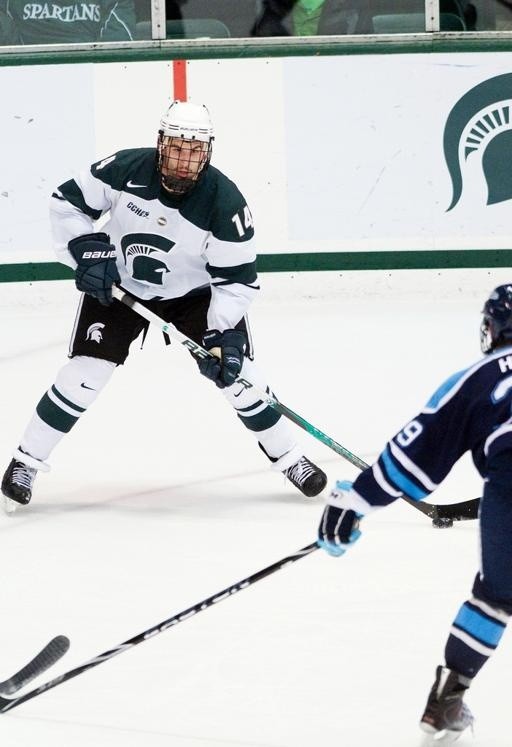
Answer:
[[481, 283, 512, 354], [155, 99, 214, 194]]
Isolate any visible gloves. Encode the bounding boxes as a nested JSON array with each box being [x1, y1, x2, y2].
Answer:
[[69, 232, 120, 308], [198, 329, 247, 388], [319, 480, 371, 557]]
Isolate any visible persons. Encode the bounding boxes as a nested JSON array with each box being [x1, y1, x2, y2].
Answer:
[[316, 284, 512, 731], [1, 99, 326, 504]]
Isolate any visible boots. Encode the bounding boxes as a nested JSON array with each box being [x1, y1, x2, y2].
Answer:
[[258, 440, 327, 497], [1, 458, 37, 505], [421, 665, 473, 734]]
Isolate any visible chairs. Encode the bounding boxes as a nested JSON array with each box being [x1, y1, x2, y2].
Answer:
[[372, 14, 466, 35], [138, 19, 231, 41]]
